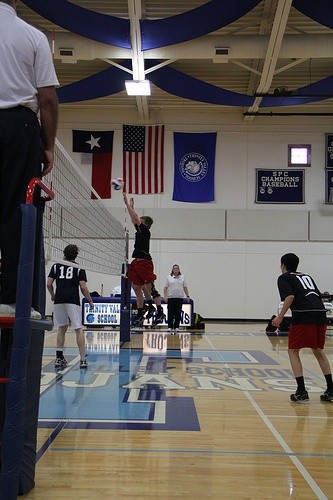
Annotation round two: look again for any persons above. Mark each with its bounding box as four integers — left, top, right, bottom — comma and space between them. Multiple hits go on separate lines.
122, 194, 164, 325
268, 252, 332, 401
0, 0, 62, 319
164, 264, 190, 332
47, 244, 94, 367
146, 285, 164, 324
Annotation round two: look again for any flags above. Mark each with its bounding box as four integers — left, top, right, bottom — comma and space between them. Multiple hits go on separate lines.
171, 130, 217, 204
71, 128, 114, 200
121, 123, 165, 195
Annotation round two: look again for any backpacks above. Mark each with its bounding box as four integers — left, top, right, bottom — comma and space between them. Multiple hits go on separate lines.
186, 313, 205, 330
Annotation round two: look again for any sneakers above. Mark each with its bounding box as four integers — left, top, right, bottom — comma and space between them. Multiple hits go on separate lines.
320, 392, 333, 402
290, 392, 309, 402
167, 328, 171, 332
0, 300, 41, 320
54, 356, 67, 366
80, 359, 88, 368
174, 328, 179, 332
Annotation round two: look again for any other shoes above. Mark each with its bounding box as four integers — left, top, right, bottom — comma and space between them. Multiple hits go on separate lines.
154, 312, 166, 325
146, 306, 156, 318
136, 305, 147, 316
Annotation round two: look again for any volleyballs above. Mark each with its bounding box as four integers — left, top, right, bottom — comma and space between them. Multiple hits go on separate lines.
112, 178, 124, 190
